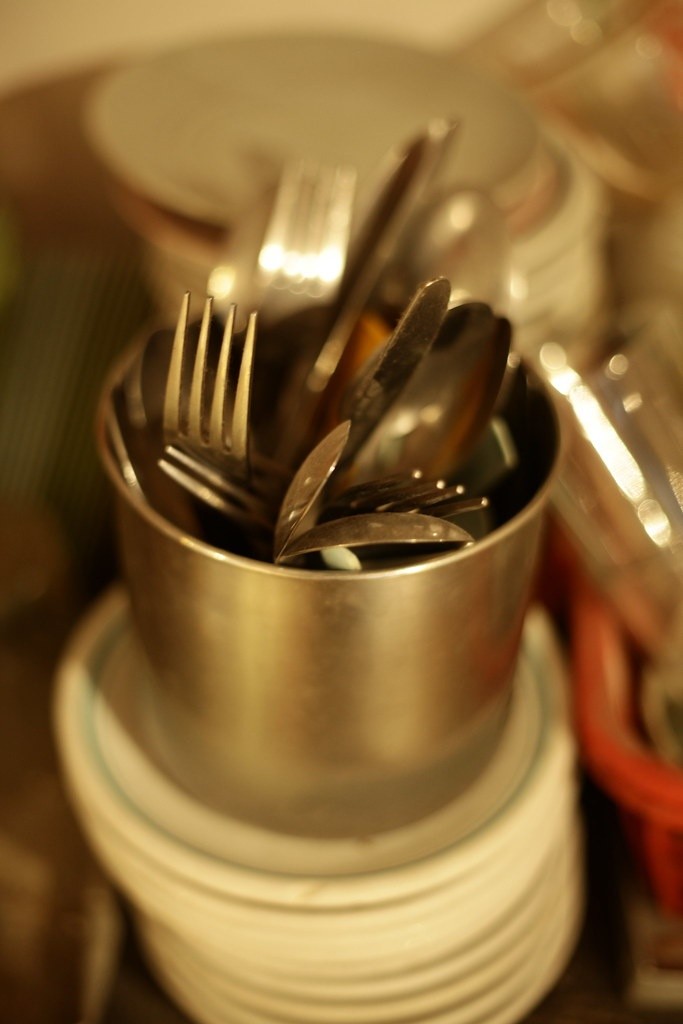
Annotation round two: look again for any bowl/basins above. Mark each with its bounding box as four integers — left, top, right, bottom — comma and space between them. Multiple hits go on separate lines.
570, 538, 680, 908
102, 157, 618, 390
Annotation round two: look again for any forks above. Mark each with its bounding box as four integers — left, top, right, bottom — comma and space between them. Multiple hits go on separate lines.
318, 467, 489, 515
247, 162, 355, 377
161, 293, 279, 542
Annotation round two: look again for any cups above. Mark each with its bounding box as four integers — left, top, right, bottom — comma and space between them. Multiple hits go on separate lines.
95, 290, 564, 836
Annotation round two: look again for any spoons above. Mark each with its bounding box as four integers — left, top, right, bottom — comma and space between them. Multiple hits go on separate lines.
339, 301, 518, 501
275, 420, 475, 569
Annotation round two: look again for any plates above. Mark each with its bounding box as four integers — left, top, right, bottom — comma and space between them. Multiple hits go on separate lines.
52, 579, 586, 1024
